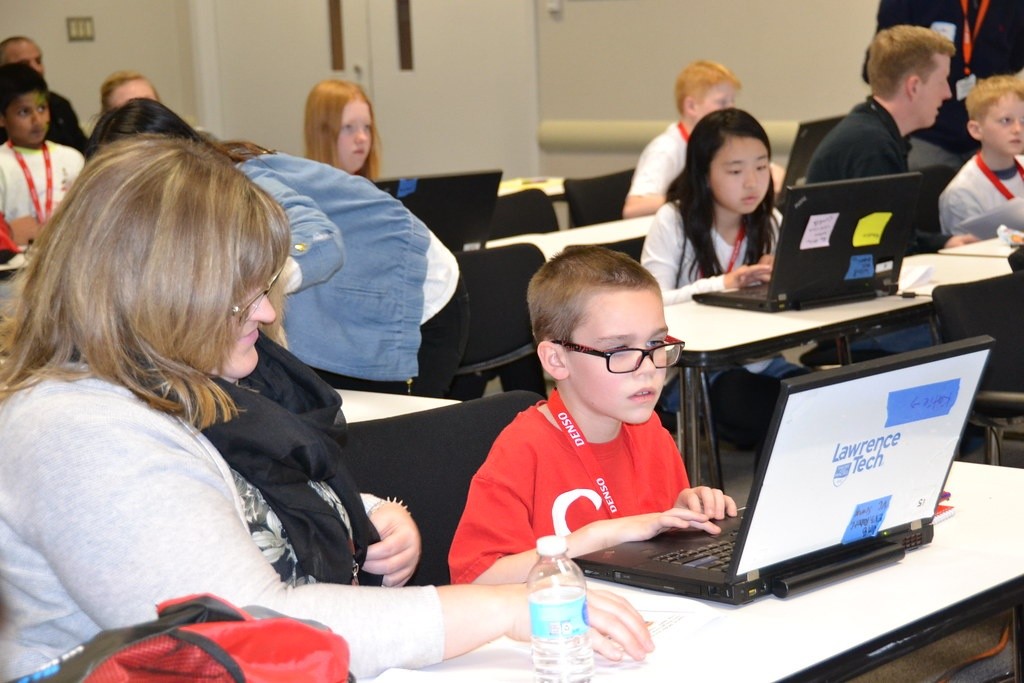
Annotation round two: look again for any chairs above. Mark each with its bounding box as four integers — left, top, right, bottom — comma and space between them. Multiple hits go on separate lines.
926, 276, 1024, 468
495, 189, 559, 238
561, 236, 646, 268
564, 167, 635, 226
449, 244, 548, 398
346, 392, 544, 587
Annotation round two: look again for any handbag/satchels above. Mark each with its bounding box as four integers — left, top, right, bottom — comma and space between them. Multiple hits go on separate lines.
8, 594, 358, 682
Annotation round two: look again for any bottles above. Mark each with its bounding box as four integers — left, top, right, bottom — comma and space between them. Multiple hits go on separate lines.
527, 535, 594, 683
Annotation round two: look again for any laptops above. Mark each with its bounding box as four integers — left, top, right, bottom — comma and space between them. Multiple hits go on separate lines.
571, 333, 996, 605
372, 171, 504, 254
691, 171, 924, 314
770, 115, 847, 212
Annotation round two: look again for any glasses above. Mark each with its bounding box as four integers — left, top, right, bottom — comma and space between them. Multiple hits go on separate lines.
226, 264, 284, 325
549, 335, 686, 375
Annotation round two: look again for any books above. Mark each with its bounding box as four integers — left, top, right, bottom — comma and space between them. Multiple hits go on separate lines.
930, 491, 956, 525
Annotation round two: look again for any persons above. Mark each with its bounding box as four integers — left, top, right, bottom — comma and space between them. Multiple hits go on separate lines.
367, 496, 411, 519
623, 60, 808, 379
304, 80, 381, 180
84, 98, 470, 398
0, 133, 653, 683
0, 35, 160, 245
806, 25, 956, 362
448, 245, 738, 584
862, 0, 1024, 190
939, 76, 1024, 241
641, 108, 793, 433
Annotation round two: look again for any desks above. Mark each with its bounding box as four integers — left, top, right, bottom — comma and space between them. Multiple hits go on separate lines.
331, 387, 461, 425
939, 236, 1023, 256
470, 213, 655, 258
893, 255, 1014, 296
661, 270, 944, 504
498, 176, 567, 199
379, 462, 1024, 683
